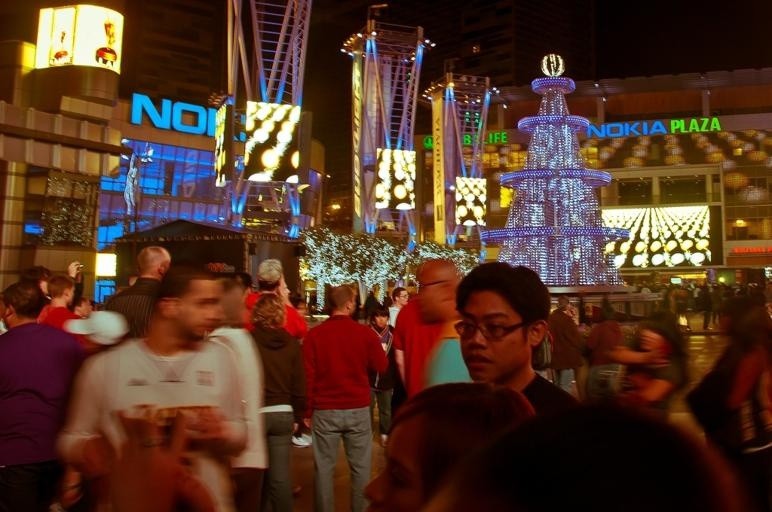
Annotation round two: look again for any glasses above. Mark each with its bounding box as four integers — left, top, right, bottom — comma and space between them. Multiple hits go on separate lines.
416, 280, 446, 288
453, 322, 527, 340
399, 294, 410, 298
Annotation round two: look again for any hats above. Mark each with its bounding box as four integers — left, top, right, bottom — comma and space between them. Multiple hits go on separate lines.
257, 258, 282, 283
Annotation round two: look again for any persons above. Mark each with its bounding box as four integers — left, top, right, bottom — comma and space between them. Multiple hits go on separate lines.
426, 407, 728, 511
623, 309, 690, 410
75, 299, 96, 319
584, 320, 680, 430
641, 273, 772, 328
243, 258, 308, 497
300, 284, 389, 512
387, 287, 409, 330
547, 296, 586, 399
454, 261, 582, 416
361, 383, 534, 511
21, 261, 85, 312
209, 288, 268, 512
37, 277, 90, 357
0, 283, 83, 512
249, 291, 307, 512
57, 269, 249, 511
370, 304, 393, 449
102, 246, 174, 347
683, 296, 772, 512
393, 258, 474, 398
282, 281, 290, 300
